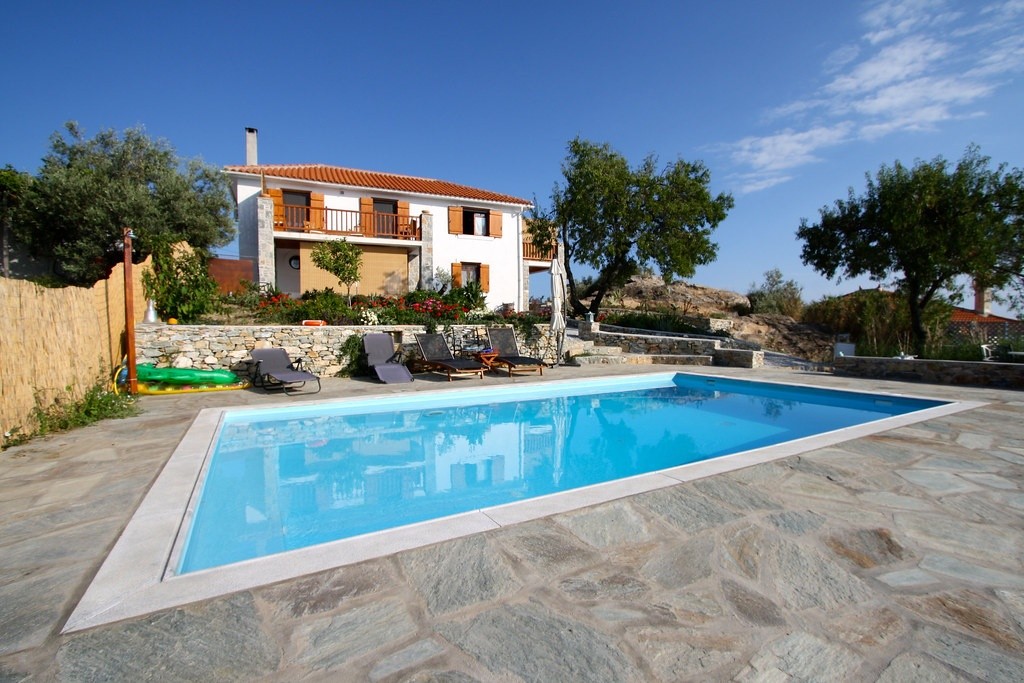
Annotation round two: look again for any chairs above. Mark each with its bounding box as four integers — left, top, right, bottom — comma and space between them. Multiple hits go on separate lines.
363, 331, 414, 383
249, 348, 322, 398
485, 327, 548, 377
413, 332, 490, 382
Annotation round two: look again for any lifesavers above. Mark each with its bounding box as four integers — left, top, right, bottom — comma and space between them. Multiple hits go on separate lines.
302, 320, 327, 326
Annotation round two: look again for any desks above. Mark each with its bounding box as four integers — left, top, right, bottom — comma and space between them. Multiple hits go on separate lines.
474, 353, 499, 376
